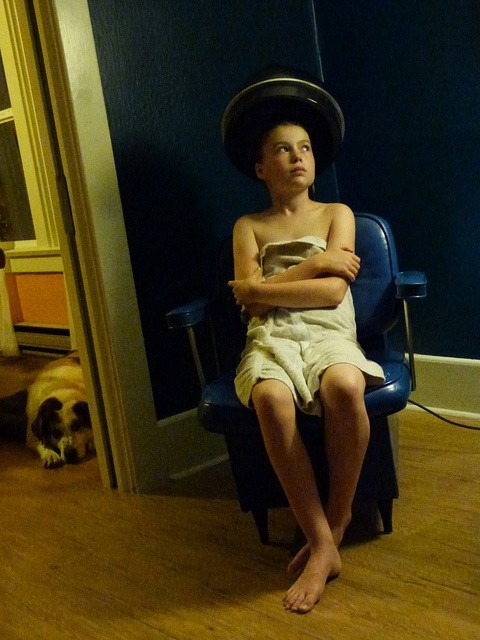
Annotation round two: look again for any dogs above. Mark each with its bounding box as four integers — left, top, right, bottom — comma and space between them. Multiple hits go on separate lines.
26, 356, 96, 469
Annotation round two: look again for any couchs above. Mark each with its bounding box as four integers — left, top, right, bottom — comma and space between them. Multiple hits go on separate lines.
171, 210, 430, 547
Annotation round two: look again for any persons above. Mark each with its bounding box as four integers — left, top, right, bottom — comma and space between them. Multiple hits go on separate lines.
228, 118, 385, 615
